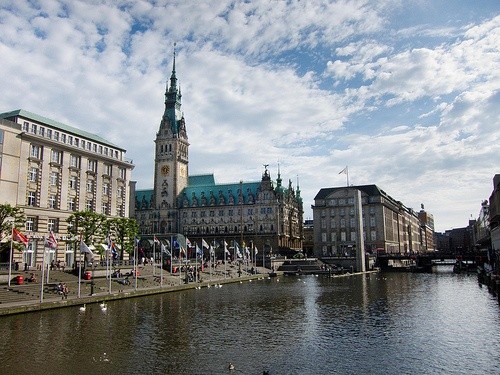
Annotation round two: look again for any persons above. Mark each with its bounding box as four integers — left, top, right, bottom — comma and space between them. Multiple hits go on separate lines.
51, 260, 60, 271
116, 269, 130, 285
173, 266, 196, 282
57, 283, 68, 299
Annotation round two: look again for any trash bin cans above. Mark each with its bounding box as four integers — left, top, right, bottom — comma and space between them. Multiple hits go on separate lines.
85, 272, 91, 279
17, 275, 24, 285
172, 267, 176, 273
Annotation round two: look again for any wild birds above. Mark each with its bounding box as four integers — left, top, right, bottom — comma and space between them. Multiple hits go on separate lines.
79, 303, 87, 312
194, 283, 223, 289
99, 301, 108, 312
239, 276, 306, 284
229, 362, 234, 371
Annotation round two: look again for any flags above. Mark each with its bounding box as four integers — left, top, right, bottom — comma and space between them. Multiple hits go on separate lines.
163, 245, 170, 255
173, 239, 179, 247
138, 243, 145, 257
202, 239, 209, 249
48, 231, 58, 248
187, 238, 192, 247
13, 227, 27, 249
112, 243, 118, 259
154, 236, 160, 245
81, 241, 93, 255
181, 247, 186, 256
196, 245, 202, 254
225, 241, 258, 258
